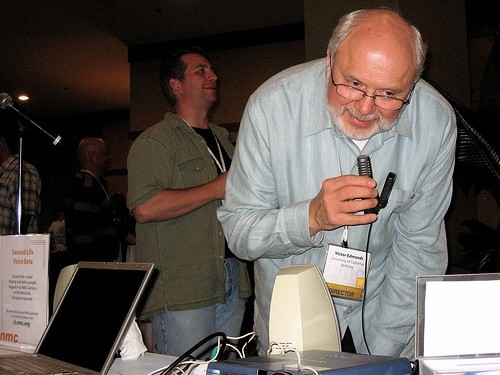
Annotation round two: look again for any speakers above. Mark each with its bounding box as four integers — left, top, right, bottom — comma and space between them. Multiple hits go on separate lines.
268, 265, 341, 353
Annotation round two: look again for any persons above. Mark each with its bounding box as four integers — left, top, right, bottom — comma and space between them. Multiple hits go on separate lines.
0, 125, 137, 265
217, 8, 457, 361
124, 47, 251, 360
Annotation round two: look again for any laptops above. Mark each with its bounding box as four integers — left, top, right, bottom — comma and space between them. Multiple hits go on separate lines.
0, 262, 155, 375
414, 272, 500, 375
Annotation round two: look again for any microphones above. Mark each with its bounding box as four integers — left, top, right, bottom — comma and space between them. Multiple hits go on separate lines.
0, 92, 63, 145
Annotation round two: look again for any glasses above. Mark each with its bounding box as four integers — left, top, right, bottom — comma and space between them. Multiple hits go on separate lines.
329, 50, 415, 110
